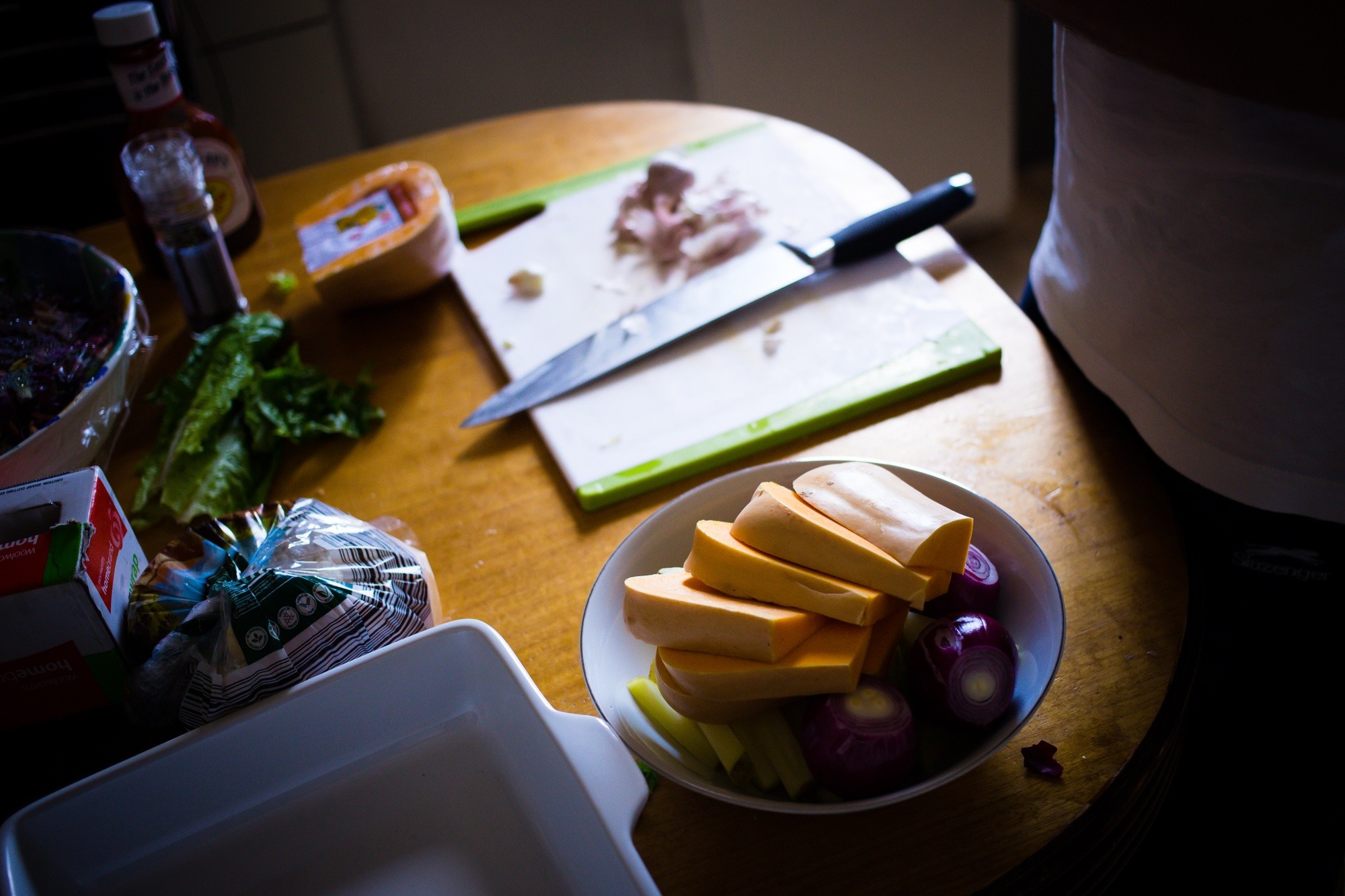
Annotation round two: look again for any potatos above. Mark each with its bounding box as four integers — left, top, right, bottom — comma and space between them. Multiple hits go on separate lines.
622, 462, 975, 727
292, 159, 460, 320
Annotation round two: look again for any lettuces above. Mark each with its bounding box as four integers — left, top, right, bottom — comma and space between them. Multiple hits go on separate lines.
133, 308, 389, 540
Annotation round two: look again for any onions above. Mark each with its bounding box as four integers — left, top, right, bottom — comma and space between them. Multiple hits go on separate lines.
798, 541, 1021, 788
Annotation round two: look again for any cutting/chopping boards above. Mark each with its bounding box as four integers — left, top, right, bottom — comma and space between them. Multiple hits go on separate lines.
444, 124, 999, 510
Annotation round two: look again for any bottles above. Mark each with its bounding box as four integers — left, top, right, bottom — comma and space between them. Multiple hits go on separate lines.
94, 2, 262, 276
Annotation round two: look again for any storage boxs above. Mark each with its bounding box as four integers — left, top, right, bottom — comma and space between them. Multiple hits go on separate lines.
0, 467, 151, 822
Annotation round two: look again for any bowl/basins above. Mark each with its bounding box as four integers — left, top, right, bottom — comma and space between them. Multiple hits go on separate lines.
1, 225, 137, 489
580, 455, 1066, 823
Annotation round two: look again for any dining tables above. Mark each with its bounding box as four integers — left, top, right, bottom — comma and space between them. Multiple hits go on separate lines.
67, 98, 1186, 896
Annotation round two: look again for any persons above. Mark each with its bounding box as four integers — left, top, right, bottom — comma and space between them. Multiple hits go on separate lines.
1010, 2, 1345, 896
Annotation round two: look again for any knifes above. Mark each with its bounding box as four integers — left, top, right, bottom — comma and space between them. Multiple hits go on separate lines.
463, 171, 977, 432
